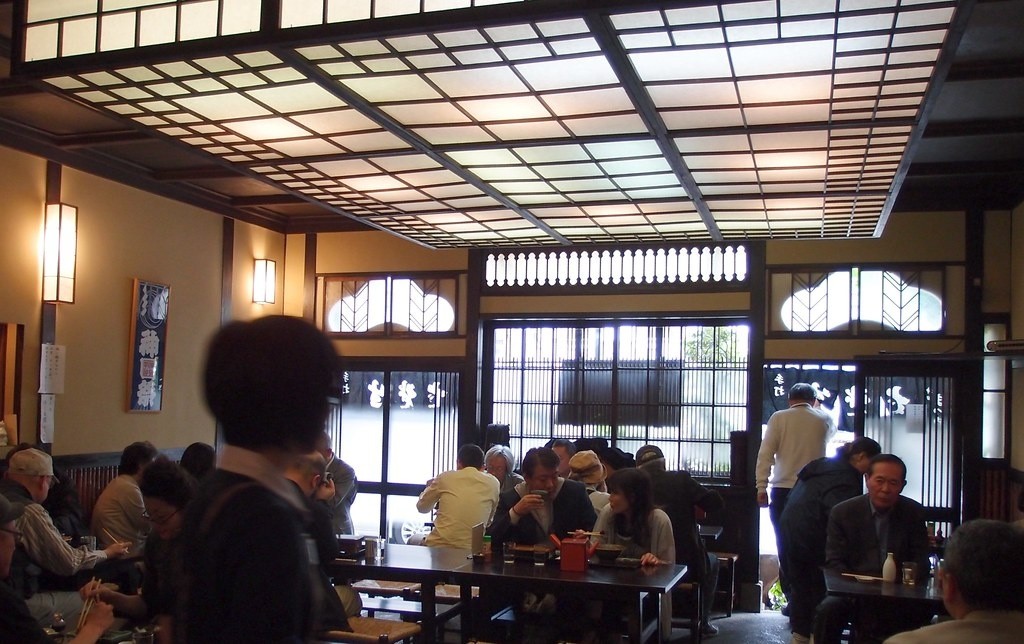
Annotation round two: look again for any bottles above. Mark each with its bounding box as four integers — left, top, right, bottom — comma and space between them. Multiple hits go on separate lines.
482, 536, 493, 561
52, 613, 66, 631
882, 553, 896, 582
365, 536, 377, 563
926, 522, 937, 543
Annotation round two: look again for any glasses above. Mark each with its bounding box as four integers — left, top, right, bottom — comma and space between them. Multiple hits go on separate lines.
323, 393, 339, 409
0, 526, 23, 542
139, 507, 180, 526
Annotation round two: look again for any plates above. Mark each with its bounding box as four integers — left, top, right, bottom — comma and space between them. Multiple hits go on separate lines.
41, 628, 58, 634
856, 576, 876, 582
67, 629, 131, 641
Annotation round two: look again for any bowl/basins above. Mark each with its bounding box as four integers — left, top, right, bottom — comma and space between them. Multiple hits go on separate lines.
595, 544, 625, 562
472, 554, 484, 563
531, 489, 548, 502
514, 546, 551, 556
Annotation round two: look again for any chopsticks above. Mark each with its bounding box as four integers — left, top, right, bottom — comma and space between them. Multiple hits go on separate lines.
843, 572, 888, 579
77, 576, 101, 629
101, 527, 130, 554
568, 531, 604, 535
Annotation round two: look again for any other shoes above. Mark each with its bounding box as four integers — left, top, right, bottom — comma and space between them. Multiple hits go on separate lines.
700, 620, 718, 637
792, 632, 809, 644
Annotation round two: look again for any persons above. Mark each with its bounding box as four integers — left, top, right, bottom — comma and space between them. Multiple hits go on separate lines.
756, 383, 837, 616
415, 439, 719, 643
1, 431, 358, 644
814, 454, 925, 644
882, 519, 1024, 644
163, 312, 346, 644
780, 437, 881, 644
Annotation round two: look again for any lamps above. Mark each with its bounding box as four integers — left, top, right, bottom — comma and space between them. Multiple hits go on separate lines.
42, 202, 78, 304
252, 259, 275, 305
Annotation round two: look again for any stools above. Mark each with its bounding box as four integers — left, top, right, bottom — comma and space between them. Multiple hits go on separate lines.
708, 551, 740, 618
320, 579, 479, 644
673, 583, 702, 644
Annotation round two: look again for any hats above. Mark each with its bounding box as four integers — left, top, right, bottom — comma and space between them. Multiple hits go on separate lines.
566, 449, 607, 484
0, 494, 25, 525
9, 449, 61, 484
788, 382, 815, 401
635, 446, 665, 470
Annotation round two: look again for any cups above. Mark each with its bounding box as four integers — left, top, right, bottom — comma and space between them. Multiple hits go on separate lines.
902, 562, 916, 585
81, 536, 96, 550
503, 542, 514, 563
534, 544, 547, 565
133, 632, 154, 644
373, 539, 385, 560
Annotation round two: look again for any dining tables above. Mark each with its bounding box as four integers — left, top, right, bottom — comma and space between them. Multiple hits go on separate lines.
333, 544, 688, 644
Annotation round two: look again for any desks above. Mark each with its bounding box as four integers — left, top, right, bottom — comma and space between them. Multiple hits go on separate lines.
821, 567, 950, 644
691, 526, 723, 644
